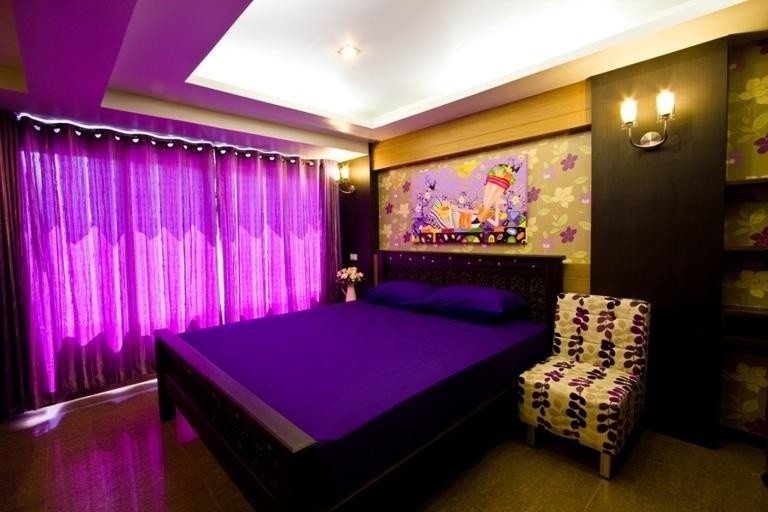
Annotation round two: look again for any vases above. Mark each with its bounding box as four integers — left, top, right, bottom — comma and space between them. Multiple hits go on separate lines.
340, 281, 357, 302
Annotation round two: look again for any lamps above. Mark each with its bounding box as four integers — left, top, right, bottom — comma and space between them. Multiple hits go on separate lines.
618, 91, 676, 149
330, 165, 354, 195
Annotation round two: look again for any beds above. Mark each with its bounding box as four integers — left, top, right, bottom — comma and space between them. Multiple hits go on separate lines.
152, 248, 566, 512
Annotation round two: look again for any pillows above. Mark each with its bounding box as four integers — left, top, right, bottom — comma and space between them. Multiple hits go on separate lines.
425, 285, 523, 322
365, 278, 443, 310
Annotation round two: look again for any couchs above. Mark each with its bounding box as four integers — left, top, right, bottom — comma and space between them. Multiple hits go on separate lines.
519, 292, 651, 481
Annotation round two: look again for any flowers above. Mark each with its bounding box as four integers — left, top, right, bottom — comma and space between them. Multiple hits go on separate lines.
332, 267, 364, 284
